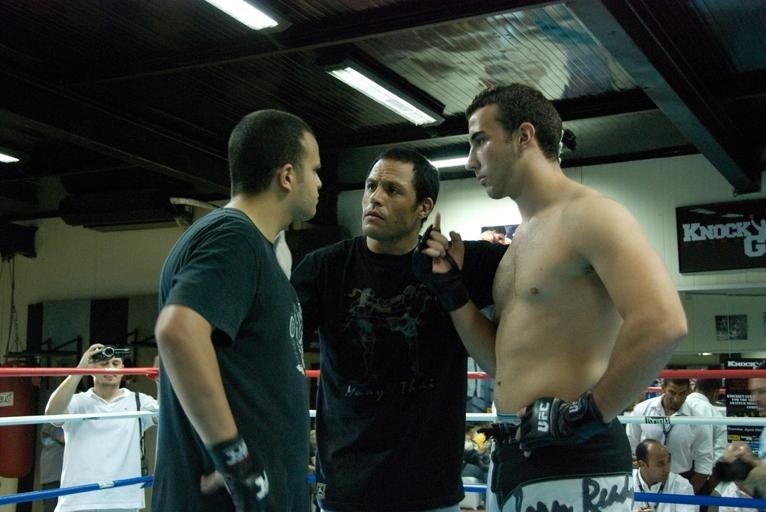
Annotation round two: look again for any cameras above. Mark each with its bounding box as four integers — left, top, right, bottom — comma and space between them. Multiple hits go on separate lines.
93, 346, 131, 361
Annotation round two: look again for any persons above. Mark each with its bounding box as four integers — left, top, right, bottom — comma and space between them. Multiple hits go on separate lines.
710, 440, 766, 499
289, 144, 517, 511
461, 418, 497, 482
303, 419, 325, 511
746, 361, 766, 460
631, 435, 702, 512
678, 361, 727, 511
409, 82, 692, 511
621, 365, 715, 496
43, 341, 162, 512
481, 225, 510, 246
152, 107, 324, 512
39, 422, 64, 511
